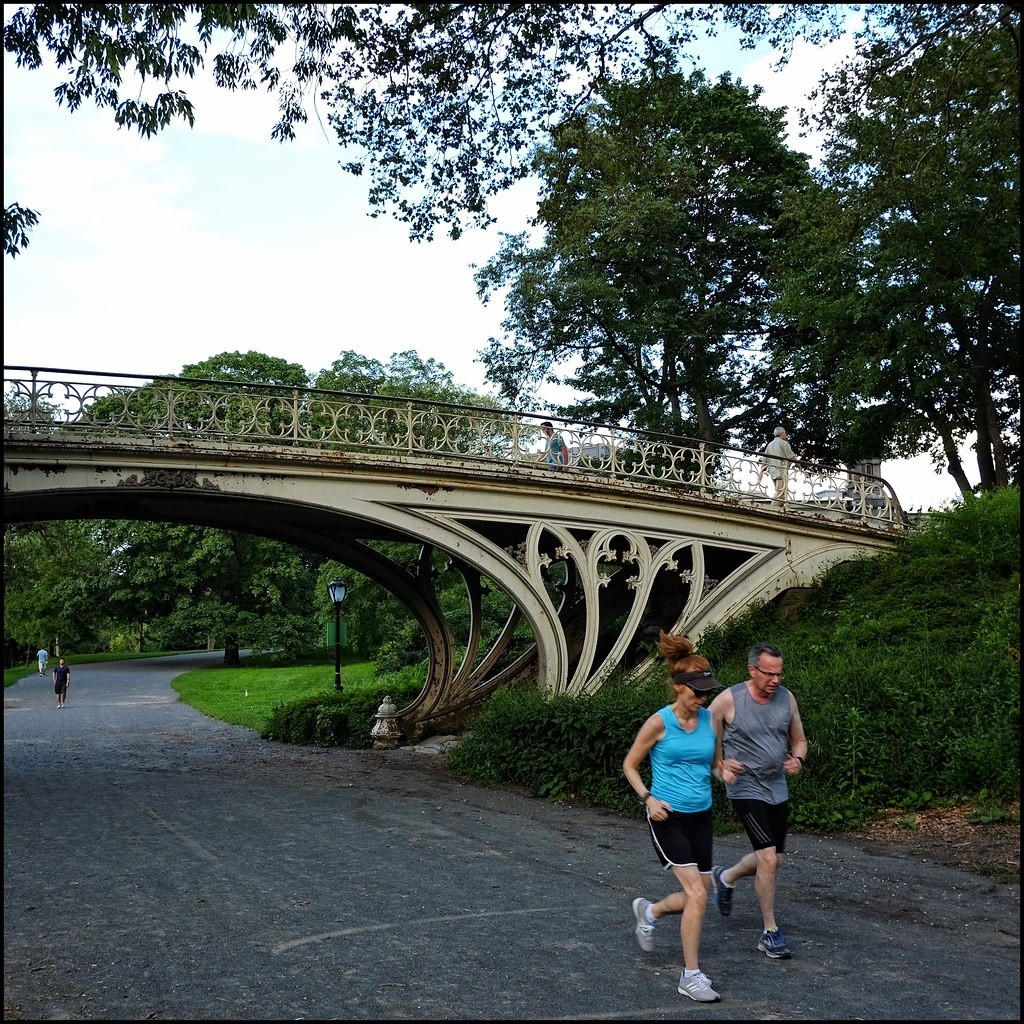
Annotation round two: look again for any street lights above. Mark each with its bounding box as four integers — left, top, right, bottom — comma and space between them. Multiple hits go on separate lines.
327, 576, 348, 691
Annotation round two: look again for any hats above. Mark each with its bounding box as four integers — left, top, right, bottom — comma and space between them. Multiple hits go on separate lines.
673, 669, 726, 691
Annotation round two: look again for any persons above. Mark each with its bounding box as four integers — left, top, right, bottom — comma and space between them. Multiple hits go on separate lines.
622, 629, 737, 1003
707, 643, 808, 959
536, 421, 569, 473
53, 658, 70, 709
36, 646, 49, 677
758, 426, 802, 508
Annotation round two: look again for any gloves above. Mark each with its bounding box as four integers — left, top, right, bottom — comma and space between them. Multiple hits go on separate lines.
46, 661, 49, 664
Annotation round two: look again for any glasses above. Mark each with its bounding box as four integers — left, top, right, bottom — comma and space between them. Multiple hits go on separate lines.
683, 683, 714, 697
752, 666, 787, 679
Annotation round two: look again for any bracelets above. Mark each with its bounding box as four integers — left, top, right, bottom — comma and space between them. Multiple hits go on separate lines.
641, 792, 652, 803
795, 757, 806, 767
67, 681, 69, 683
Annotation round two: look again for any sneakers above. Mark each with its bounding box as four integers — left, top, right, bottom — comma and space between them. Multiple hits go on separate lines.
677, 971, 721, 1002
710, 865, 735, 917
632, 897, 657, 952
757, 930, 792, 960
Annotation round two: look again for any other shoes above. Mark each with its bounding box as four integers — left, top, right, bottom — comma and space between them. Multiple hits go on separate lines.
57, 705, 65, 709
39, 674, 44, 677
